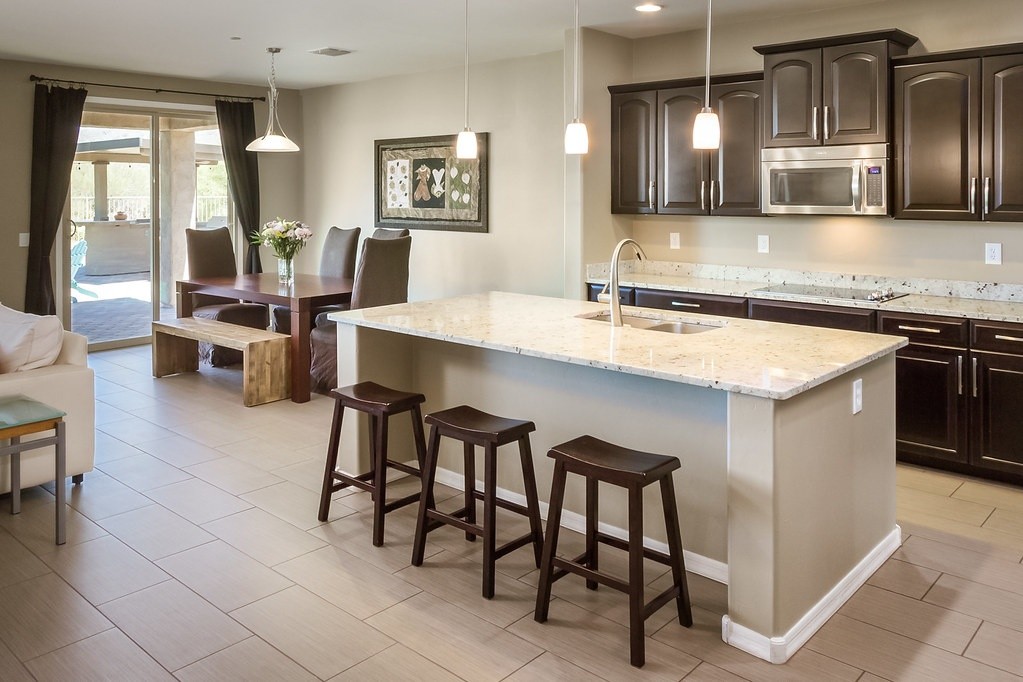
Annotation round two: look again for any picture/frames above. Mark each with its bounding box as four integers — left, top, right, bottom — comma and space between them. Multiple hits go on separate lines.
371, 131, 491, 233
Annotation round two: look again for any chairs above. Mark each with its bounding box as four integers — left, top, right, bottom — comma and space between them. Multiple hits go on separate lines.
310, 235, 413, 393
71, 239, 99, 303
272, 227, 360, 334
184, 225, 267, 368
272, 227, 411, 335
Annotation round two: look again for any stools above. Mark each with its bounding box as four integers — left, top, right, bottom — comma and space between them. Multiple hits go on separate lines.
402, 402, 544, 600
312, 378, 426, 545
533, 433, 693, 668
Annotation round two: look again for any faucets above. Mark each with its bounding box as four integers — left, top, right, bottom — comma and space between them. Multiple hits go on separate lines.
610, 238, 647, 327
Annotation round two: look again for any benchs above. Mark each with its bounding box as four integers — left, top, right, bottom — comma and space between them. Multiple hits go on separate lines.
152, 319, 291, 407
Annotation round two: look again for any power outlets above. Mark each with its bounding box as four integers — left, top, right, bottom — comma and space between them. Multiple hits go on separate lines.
669, 232, 682, 251
985, 243, 1003, 264
851, 379, 867, 415
757, 234, 770, 253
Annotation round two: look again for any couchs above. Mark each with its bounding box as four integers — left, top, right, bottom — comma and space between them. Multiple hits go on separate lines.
0, 299, 98, 498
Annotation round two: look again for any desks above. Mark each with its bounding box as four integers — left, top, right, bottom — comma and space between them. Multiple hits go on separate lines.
170, 267, 354, 404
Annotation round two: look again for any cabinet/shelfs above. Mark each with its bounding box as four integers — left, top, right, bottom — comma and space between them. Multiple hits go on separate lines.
588, 284, 1022, 483
753, 30, 920, 145
606, 86, 658, 214
886, 44, 1023, 223
655, 72, 764, 218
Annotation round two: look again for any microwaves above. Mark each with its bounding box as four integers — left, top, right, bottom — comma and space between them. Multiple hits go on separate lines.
759, 143, 894, 217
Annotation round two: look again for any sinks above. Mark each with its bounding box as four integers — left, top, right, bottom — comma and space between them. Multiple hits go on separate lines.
585, 313, 661, 330
644, 322, 723, 334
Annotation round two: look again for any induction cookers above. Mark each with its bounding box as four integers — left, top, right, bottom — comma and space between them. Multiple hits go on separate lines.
750, 283, 908, 310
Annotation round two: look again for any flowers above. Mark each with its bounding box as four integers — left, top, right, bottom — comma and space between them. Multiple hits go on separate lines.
253, 212, 311, 253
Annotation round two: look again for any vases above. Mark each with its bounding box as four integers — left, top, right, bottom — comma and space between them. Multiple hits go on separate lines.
279, 251, 295, 279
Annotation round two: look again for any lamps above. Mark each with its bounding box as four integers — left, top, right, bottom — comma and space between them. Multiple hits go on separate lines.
456, 1, 477, 161
693, 1, 720, 149
560, 0, 591, 155
246, 48, 299, 153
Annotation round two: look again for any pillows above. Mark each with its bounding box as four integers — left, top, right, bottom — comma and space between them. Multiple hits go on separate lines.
0, 303, 66, 372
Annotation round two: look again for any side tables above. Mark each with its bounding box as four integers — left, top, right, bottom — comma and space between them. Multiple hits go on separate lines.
0, 397, 70, 555
